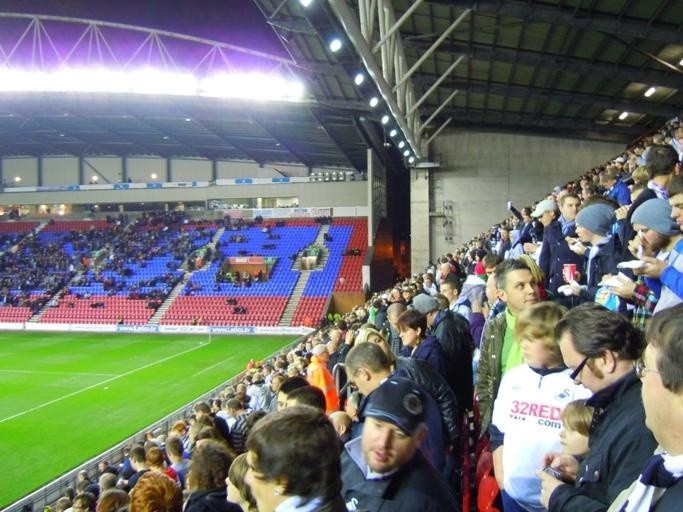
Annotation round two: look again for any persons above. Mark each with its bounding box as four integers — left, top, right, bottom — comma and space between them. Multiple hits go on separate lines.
1, 117, 682, 512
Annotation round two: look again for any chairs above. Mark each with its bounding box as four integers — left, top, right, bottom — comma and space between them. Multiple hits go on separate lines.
462, 391, 501, 512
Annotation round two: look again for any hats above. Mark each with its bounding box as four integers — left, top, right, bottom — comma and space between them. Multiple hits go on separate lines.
410, 293, 441, 316
529, 199, 558, 220
360, 375, 429, 439
630, 198, 680, 238
574, 203, 616, 239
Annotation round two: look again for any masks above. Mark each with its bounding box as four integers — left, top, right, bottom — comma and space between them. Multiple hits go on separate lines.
527, 365, 568, 379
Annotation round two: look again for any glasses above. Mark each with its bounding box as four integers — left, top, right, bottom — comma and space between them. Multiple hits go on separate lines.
634, 355, 673, 383
570, 356, 589, 383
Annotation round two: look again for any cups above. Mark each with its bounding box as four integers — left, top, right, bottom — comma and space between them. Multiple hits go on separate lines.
563, 263, 576, 284
